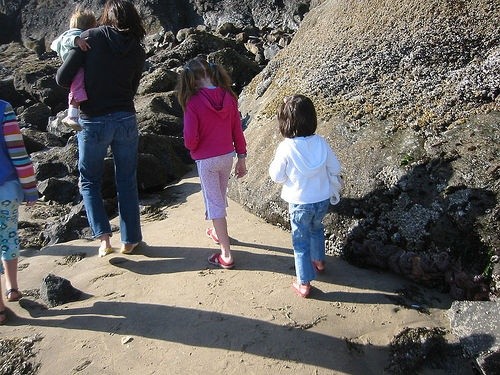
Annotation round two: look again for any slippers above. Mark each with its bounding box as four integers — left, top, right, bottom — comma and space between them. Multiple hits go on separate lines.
208, 254, 235, 268
206, 228, 220, 243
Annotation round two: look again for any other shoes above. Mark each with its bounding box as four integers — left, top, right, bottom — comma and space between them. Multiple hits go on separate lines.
120, 242, 138, 253
293, 280, 310, 295
312, 259, 325, 271
98, 247, 112, 255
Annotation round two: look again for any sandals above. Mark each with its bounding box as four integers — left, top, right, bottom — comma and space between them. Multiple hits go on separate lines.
7, 288, 22, 301
0, 308, 7, 324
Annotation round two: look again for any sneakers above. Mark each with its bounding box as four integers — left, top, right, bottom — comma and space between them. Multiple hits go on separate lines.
61, 117, 82, 131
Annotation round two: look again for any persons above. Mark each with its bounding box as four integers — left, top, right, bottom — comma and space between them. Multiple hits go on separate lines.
56, 10, 97, 132
0, 98, 38, 326
178, 57, 248, 269
55, 0, 147, 257
269, 94, 342, 298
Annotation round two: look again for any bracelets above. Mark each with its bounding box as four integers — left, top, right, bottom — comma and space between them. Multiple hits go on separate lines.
237, 153, 247, 159
